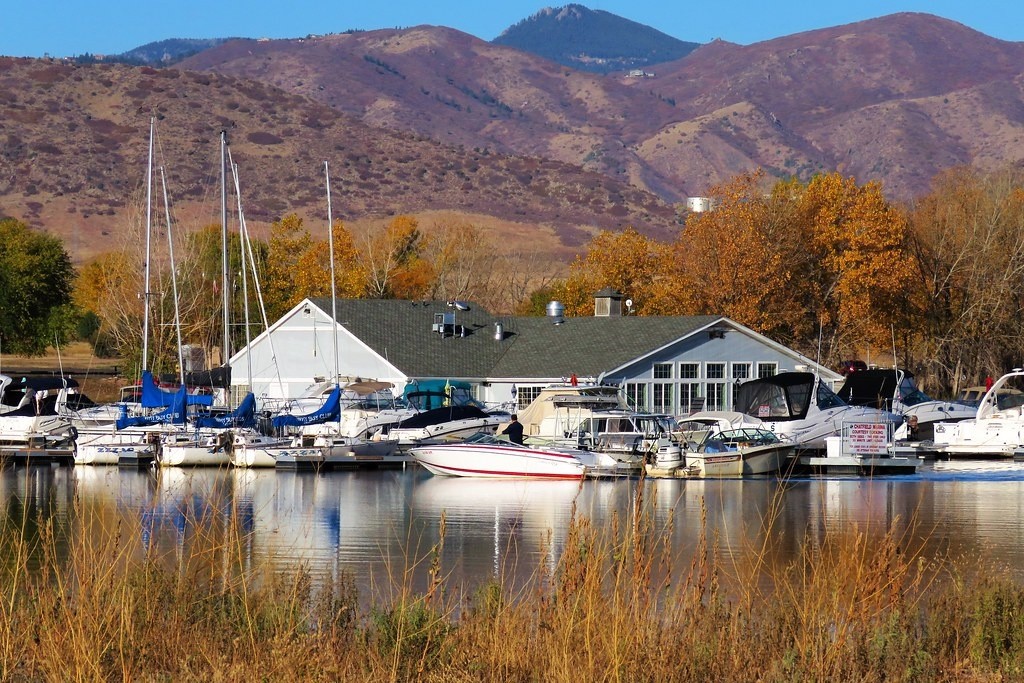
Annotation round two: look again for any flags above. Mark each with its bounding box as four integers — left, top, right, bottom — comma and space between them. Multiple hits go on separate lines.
893, 421, 908, 440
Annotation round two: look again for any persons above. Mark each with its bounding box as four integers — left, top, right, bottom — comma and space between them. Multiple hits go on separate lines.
984, 375, 993, 393
570, 372, 578, 387
511, 383, 517, 397
496, 414, 526, 446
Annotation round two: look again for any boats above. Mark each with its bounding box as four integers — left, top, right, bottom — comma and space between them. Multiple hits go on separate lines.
1, 115, 1024, 483
